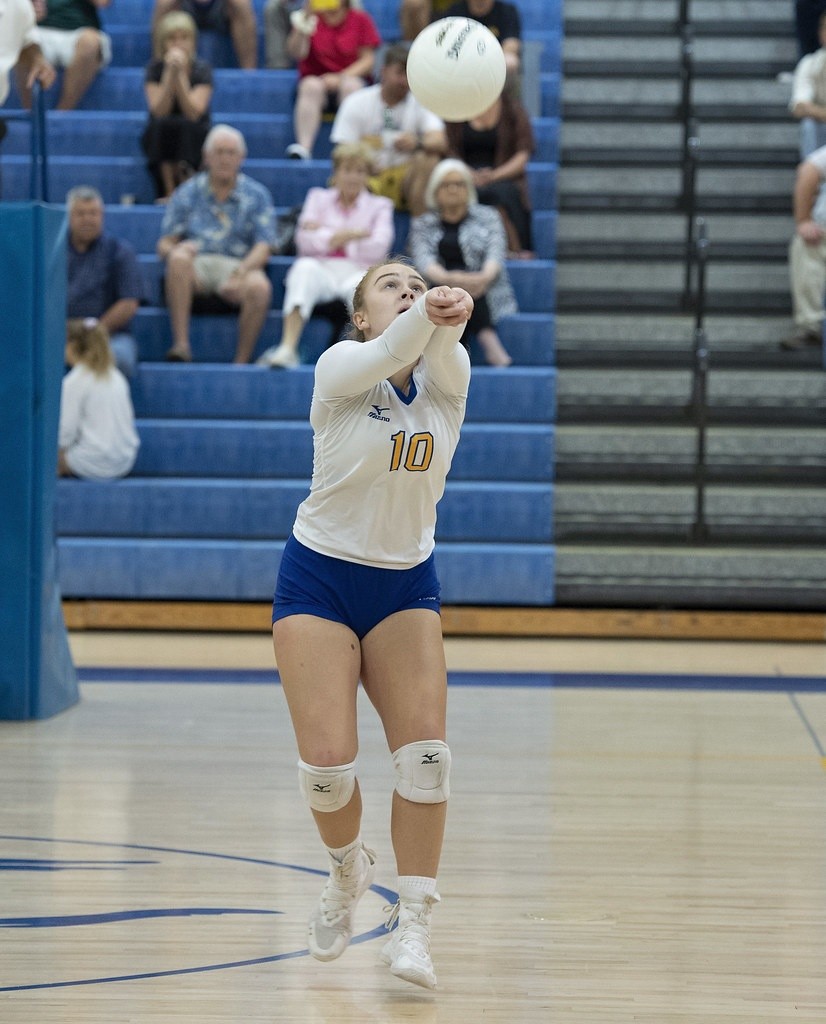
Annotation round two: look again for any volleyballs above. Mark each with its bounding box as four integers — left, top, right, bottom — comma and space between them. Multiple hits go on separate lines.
406, 16, 506, 122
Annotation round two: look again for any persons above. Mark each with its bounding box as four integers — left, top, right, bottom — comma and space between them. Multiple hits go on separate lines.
782, 0, 826, 350
0, 0, 114, 142
271, 259, 472, 991
57, 184, 149, 480
139, 0, 258, 205
155, 123, 275, 364
262, 0, 536, 369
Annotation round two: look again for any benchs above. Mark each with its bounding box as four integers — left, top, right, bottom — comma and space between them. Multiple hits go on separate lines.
0, 0, 562, 610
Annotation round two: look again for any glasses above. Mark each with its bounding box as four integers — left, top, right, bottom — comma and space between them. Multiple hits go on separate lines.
440, 181, 466, 188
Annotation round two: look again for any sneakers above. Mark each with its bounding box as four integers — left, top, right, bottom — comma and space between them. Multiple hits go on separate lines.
380, 887, 441, 991
307, 841, 378, 963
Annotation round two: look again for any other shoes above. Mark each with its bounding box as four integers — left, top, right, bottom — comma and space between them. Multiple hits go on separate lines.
284, 144, 309, 161
165, 352, 192, 363
270, 347, 300, 370
780, 330, 824, 351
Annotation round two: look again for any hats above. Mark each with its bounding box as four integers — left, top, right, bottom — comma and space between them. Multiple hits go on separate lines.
310, 0, 340, 11
